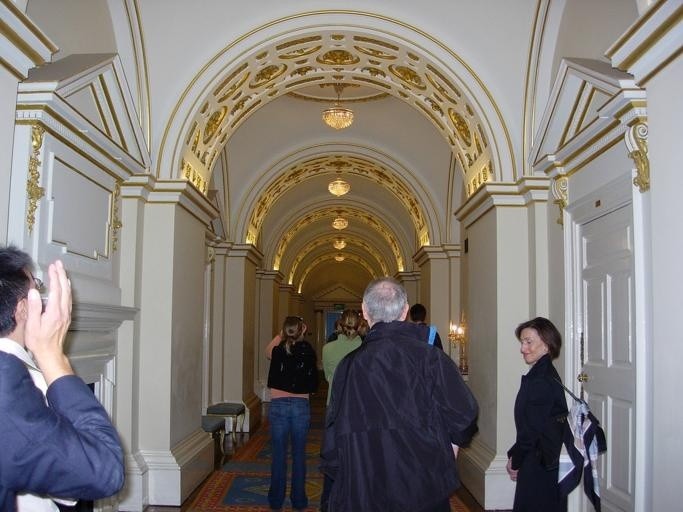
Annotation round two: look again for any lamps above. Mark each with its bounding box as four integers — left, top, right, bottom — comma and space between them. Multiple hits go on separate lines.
322, 85, 356, 133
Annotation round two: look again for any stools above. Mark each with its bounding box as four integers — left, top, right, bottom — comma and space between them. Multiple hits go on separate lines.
203, 400, 246, 456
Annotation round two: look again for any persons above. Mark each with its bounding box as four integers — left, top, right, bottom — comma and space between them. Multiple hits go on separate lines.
410, 304, 443, 351
322, 309, 363, 406
327, 319, 342, 343
358, 318, 369, 342
0, 241, 125, 512
506, 316, 569, 512
319, 276, 479, 511
265, 316, 318, 510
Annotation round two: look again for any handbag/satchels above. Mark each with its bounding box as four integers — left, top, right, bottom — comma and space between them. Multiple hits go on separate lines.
538, 404, 607, 472
